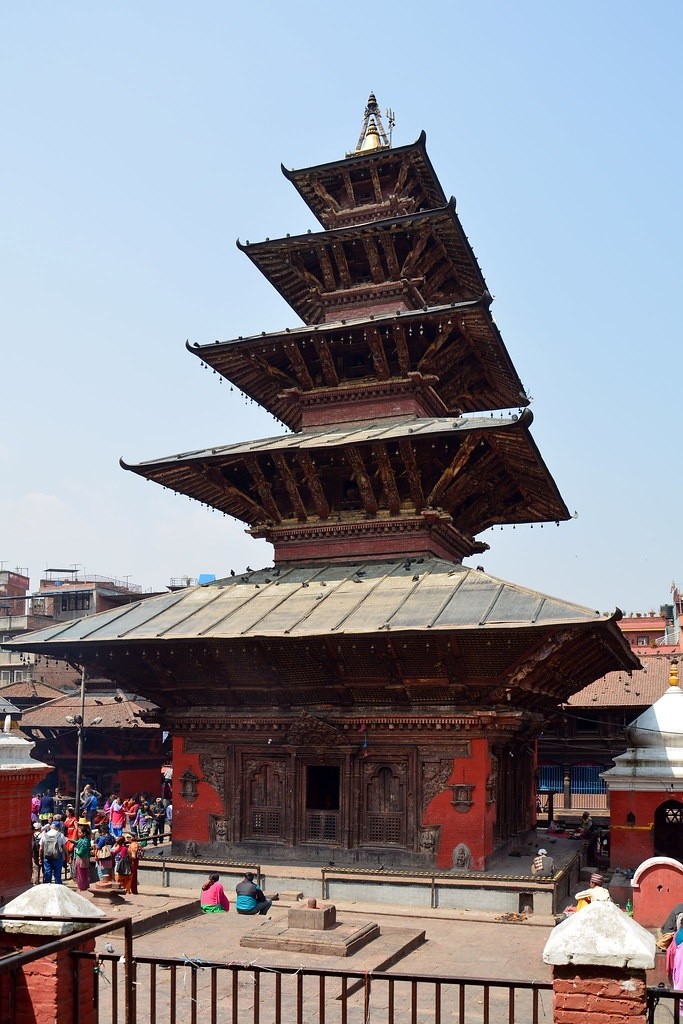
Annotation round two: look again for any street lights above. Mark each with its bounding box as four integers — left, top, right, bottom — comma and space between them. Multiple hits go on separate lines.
65, 715, 104, 880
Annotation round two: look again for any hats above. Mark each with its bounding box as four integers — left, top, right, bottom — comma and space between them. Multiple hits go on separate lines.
591, 874, 605, 885
584, 811, 590, 816
75, 818, 91, 825
52, 821, 61, 829
538, 849, 547, 856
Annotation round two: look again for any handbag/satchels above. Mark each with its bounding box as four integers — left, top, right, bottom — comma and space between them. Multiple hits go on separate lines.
137, 845, 144, 859
97, 844, 111, 860
116, 859, 131, 874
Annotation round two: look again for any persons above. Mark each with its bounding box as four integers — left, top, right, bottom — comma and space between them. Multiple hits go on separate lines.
200, 874, 229, 913
531, 849, 557, 877
31, 784, 172, 894
236, 872, 272, 915
575, 874, 611, 911
656, 902, 683, 1017
457, 848, 465, 867
580, 812, 594, 836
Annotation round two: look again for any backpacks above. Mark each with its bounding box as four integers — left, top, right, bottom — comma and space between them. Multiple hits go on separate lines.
43, 832, 61, 859
33, 832, 41, 858
